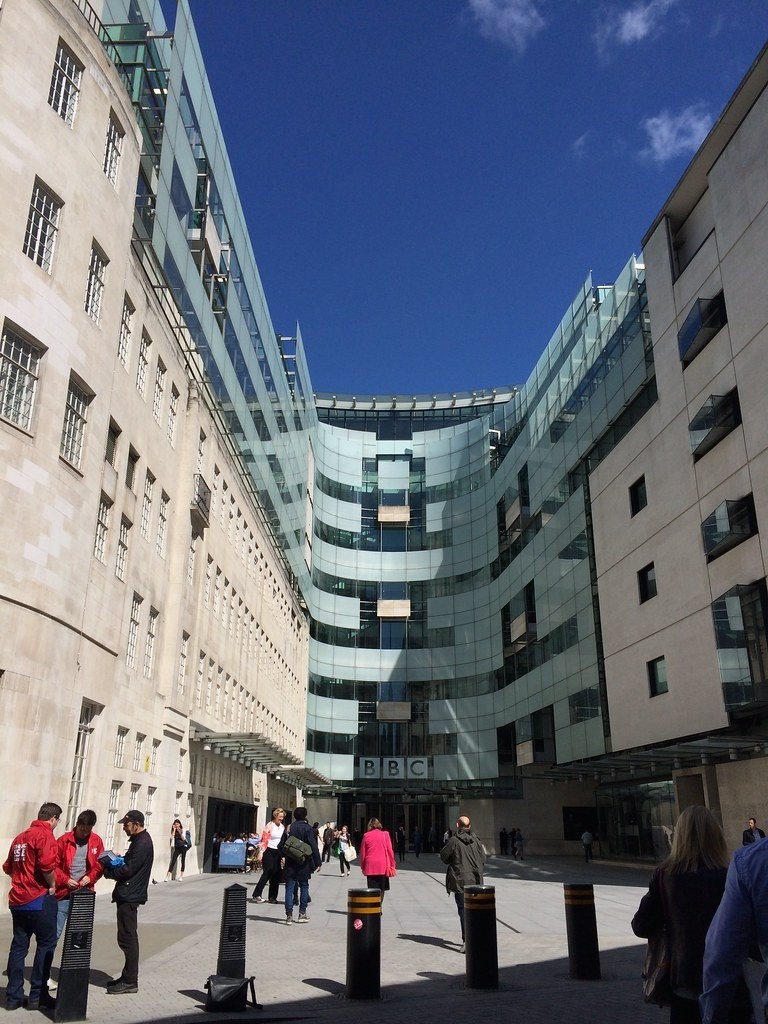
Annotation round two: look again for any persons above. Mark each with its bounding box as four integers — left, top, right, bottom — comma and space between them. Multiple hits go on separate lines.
443, 827, 452, 844
321, 822, 338, 863
164, 819, 188, 882
312, 822, 324, 847
103, 810, 155, 992
3, 802, 63, 1011
509, 828, 524, 860
441, 818, 487, 953
698, 838, 768, 1024
631, 805, 728, 1024
276, 807, 321, 925
252, 808, 286, 904
360, 817, 397, 916
48, 810, 103, 991
213, 830, 259, 874
742, 817, 766, 846
581, 829, 593, 861
428, 827, 438, 853
395, 823, 405, 862
500, 828, 508, 855
335, 825, 352, 877
411, 825, 423, 858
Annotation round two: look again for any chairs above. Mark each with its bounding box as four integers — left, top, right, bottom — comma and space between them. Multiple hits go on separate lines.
246, 844, 262, 873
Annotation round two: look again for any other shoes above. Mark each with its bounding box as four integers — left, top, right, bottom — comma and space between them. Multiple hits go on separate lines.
340, 873, 344, 877
178, 878, 182, 881
287, 916, 292, 925
347, 869, 350, 875
164, 877, 169, 881
307, 896, 311, 902
461, 943, 466, 953
299, 914, 310, 922
292, 899, 299, 905
47, 978, 59, 990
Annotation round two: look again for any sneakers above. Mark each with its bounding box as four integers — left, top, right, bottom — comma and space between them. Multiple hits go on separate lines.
252, 895, 265, 903
6, 995, 28, 1010
107, 977, 121, 986
268, 897, 278, 903
28, 994, 57, 1009
107, 980, 138, 993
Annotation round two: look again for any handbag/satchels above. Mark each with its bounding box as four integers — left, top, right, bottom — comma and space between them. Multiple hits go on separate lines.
642, 933, 688, 1004
177, 832, 191, 850
282, 836, 312, 865
343, 847, 357, 862
386, 866, 396, 877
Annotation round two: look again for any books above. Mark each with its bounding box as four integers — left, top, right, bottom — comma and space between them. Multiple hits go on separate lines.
97, 849, 126, 868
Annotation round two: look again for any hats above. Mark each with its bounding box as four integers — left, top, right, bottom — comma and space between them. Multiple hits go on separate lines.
118, 810, 144, 823
